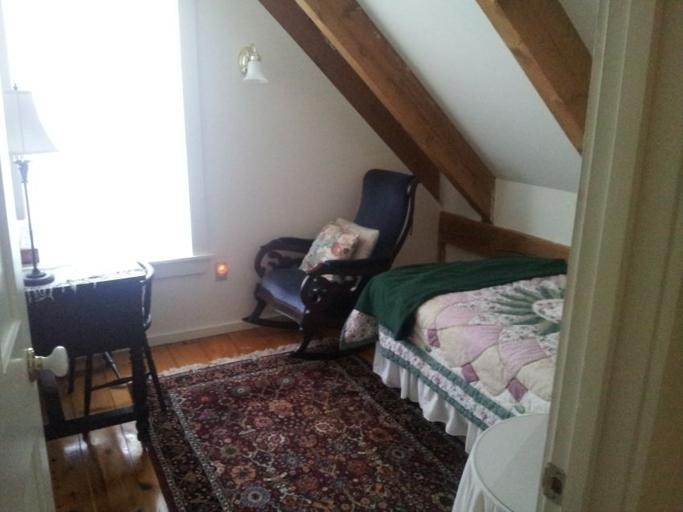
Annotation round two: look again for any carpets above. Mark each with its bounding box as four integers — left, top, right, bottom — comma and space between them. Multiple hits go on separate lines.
126, 336, 470, 512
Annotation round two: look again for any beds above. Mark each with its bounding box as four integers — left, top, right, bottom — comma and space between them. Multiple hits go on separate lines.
372, 252, 571, 455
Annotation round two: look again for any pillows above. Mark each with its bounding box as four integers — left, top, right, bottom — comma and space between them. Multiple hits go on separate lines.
334, 217, 381, 283
298, 221, 360, 282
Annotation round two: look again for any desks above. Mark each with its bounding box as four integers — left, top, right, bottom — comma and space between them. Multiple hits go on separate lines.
21, 252, 152, 442
451, 414, 549, 512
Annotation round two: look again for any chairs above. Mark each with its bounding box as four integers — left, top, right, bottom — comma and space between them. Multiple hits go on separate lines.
242, 169, 422, 359
64, 259, 168, 440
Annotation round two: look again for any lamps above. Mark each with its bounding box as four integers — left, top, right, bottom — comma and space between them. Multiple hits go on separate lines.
237, 44, 269, 84
3, 84, 57, 286
